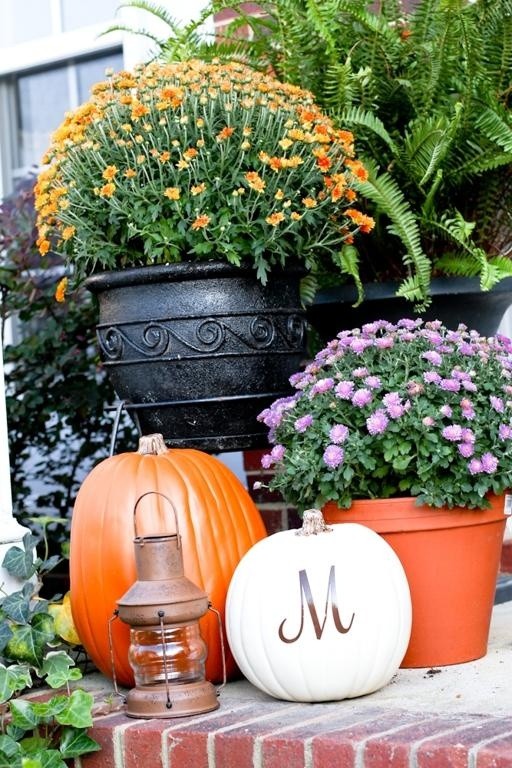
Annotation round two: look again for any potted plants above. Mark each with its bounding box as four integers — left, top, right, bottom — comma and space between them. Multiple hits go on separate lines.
98, 0, 512, 338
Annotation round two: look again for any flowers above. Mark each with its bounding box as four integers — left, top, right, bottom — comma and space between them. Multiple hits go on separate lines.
256, 319, 512, 512
34, 59, 377, 300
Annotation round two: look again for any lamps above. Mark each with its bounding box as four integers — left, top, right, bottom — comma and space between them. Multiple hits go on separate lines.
107, 491, 227, 719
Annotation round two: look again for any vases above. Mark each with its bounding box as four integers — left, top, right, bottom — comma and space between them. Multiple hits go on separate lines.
84, 261, 305, 452
319, 490, 512, 668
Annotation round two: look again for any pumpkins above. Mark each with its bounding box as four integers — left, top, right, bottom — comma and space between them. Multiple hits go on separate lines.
221, 511, 415, 704
65, 432, 271, 687
